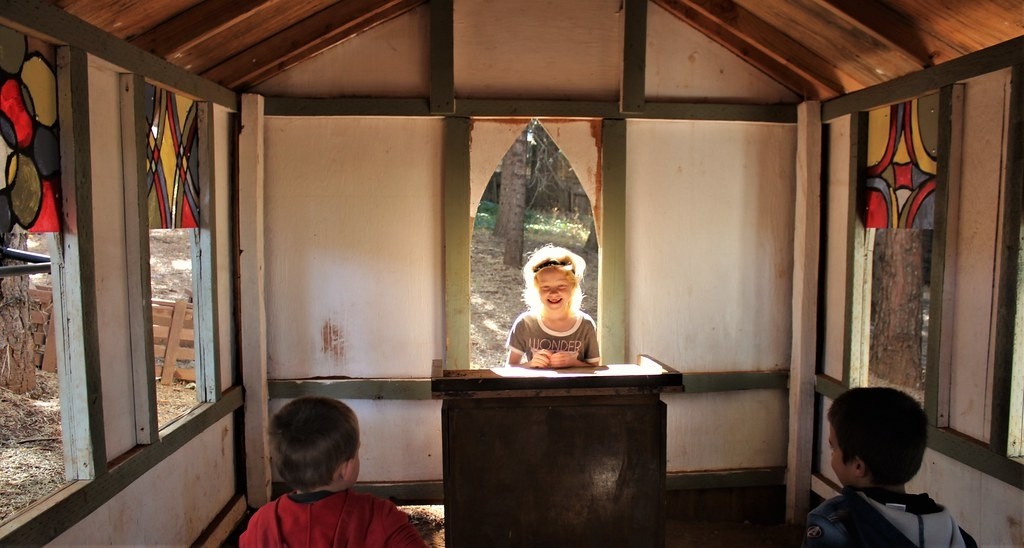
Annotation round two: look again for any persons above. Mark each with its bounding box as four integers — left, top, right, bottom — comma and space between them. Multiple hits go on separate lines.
238, 396, 428, 548
504, 248, 600, 369
805, 387, 979, 548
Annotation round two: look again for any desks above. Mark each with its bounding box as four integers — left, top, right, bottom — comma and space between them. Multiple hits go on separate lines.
431, 354, 684, 548
